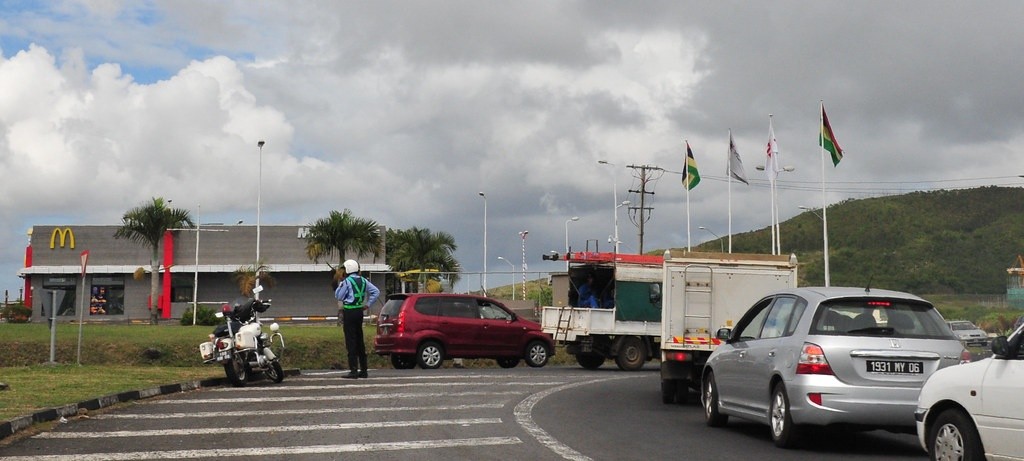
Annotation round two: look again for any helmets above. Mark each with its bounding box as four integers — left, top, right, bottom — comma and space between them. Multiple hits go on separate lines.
341, 259, 360, 274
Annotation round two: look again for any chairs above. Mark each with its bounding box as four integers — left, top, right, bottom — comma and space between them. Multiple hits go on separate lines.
824, 312, 916, 335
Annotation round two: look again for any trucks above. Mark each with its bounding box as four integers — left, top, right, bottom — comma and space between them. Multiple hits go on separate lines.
539, 250, 669, 373
659, 248, 799, 410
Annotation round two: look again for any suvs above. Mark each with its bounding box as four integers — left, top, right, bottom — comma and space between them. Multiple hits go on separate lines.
373, 291, 557, 371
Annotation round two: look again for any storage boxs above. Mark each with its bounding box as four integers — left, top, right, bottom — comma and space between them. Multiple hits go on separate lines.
200, 342, 214, 359
234, 332, 257, 348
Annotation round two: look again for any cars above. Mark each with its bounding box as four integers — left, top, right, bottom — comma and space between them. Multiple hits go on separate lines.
913, 322, 1024, 461
699, 285, 971, 448
946, 320, 992, 345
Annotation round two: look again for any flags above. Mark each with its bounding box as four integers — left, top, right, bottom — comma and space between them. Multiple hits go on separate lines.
681, 144, 700, 190
818, 104, 845, 167
763, 120, 779, 186
726, 134, 749, 185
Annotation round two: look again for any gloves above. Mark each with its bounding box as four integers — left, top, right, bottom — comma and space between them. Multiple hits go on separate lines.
363, 305, 368, 310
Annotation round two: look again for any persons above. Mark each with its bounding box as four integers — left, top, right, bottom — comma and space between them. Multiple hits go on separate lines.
334, 259, 380, 379
577, 274, 599, 308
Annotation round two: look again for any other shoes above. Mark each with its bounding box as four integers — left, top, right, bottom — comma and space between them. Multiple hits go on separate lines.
342, 371, 358, 379
358, 369, 367, 378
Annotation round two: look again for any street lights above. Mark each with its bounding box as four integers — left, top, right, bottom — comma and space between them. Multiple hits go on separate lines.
30, 286, 34, 310
564, 215, 580, 258
498, 256, 516, 301
598, 159, 630, 255
756, 164, 798, 258
478, 192, 488, 319
255, 140, 264, 323
798, 206, 835, 289
698, 226, 726, 257
518, 230, 531, 300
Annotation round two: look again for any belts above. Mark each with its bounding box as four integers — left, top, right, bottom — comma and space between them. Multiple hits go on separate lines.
344, 305, 363, 309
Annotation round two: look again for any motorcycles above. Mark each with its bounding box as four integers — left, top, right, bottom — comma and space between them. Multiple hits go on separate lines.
198, 297, 285, 387
